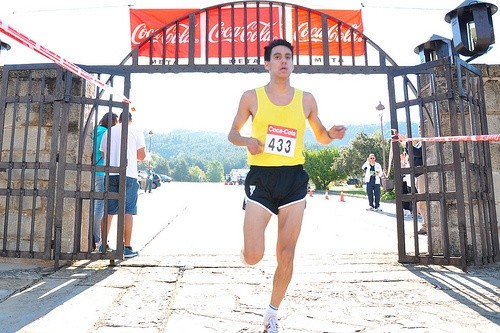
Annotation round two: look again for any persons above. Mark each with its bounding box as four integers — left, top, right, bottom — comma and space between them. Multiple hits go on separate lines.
400, 124, 427, 235
98, 110, 148, 258
144, 166, 155, 193
227, 174, 243, 185
227, 39, 349, 333
362, 154, 383, 212
92, 111, 118, 250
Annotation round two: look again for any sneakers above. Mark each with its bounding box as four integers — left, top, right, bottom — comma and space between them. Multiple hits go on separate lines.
264, 314, 279, 333
124, 247, 138, 258
99, 244, 112, 253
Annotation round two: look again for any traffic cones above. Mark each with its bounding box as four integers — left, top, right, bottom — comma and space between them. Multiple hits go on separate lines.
324, 189, 329, 199
339, 190, 346, 202
309, 191, 314, 197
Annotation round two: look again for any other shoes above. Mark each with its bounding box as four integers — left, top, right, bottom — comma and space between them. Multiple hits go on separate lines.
418, 215, 422, 219
375, 208, 382, 212
366, 207, 374, 211
417, 229, 427, 234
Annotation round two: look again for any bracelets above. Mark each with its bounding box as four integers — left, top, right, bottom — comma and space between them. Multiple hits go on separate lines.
327, 132, 335, 139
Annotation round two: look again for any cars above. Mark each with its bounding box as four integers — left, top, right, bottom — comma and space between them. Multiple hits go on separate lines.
138, 173, 173, 190
224, 168, 251, 185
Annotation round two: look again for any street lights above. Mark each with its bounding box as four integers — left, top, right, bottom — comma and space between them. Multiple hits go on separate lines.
148, 130, 154, 169
375, 100, 385, 174
